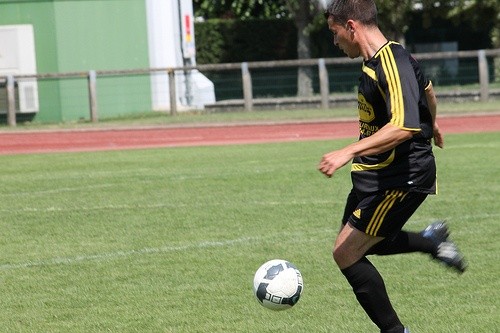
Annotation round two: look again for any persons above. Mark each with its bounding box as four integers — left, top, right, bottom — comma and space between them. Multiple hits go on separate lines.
317, 0, 468, 333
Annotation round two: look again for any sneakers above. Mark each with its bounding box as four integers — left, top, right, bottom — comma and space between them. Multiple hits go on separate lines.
421, 222, 466, 274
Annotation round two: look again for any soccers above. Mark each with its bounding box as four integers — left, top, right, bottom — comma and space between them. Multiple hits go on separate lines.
253, 259, 304, 311
